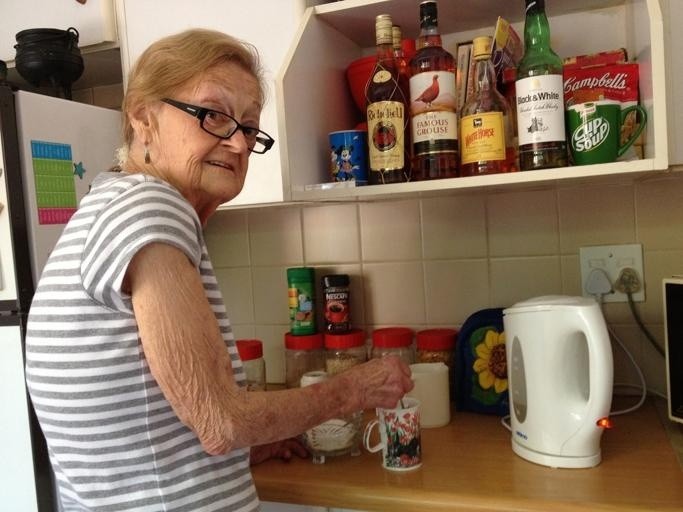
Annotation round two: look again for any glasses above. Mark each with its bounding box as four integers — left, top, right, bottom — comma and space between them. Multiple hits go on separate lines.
159, 99, 274, 154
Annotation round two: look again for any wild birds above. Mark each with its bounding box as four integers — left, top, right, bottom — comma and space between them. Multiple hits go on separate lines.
414, 73, 440, 109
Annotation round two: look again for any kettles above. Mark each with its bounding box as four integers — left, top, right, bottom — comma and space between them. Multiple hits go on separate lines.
500, 291, 617, 459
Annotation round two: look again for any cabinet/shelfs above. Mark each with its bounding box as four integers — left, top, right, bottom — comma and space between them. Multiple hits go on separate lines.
1, 0, 668, 212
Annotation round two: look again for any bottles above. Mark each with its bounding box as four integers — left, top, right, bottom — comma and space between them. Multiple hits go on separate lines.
235, 337, 268, 395
362, 9, 411, 186
323, 329, 370, 377
282, 332, 328, 391
414, 326, 461, 368
368, 326, 413, 367
320, 272, 353, 334
407, 0, 460, 184
299, 371, 327, 389
512, 0, 570, 173
285, 264, 317, 337
456, 34, 519, 179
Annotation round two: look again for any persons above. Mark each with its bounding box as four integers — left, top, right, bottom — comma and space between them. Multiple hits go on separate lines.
23, 23, 417, 512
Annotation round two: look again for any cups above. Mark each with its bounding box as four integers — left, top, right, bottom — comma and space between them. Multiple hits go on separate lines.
400, 362, 451, 429
362, 397, 423, 473
327, 127, 370, 187
563, 99, 648, 167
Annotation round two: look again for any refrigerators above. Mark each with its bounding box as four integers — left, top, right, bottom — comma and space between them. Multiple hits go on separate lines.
0, 56, 155, 511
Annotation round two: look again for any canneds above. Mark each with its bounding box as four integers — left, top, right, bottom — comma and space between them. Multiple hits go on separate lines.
323, 274, 350, 334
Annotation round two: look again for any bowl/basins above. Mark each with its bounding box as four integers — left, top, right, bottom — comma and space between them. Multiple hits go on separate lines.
296, 410, 367, 461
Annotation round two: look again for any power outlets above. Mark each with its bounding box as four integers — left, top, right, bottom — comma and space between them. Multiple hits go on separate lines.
581, 244, 648, 308
